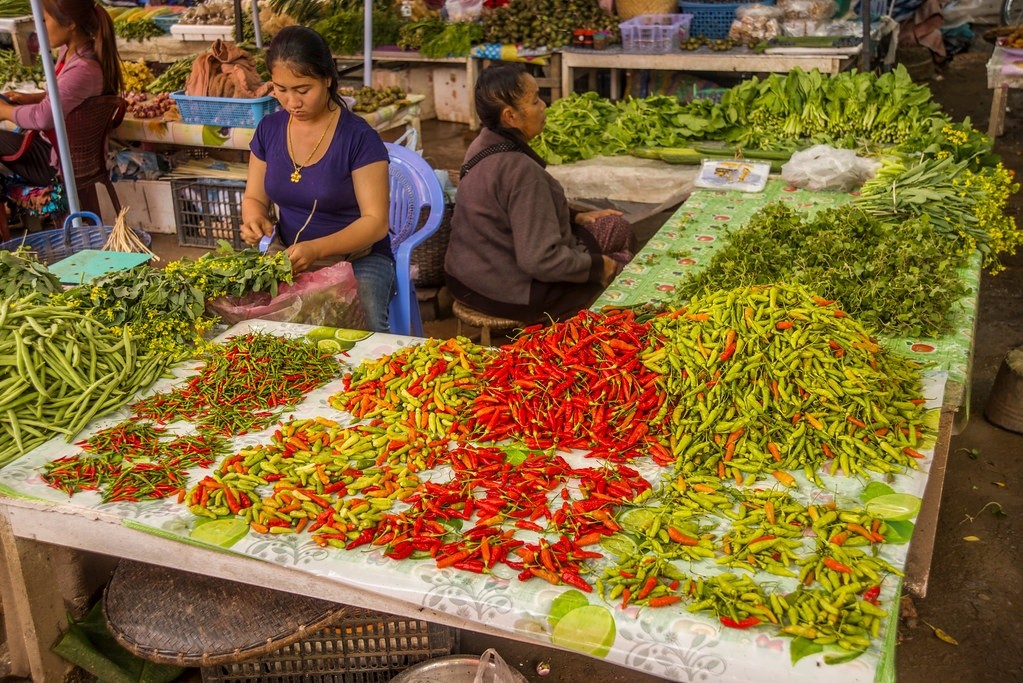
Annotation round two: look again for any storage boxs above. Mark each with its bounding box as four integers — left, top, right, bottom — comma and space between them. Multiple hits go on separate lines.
678, 0, 776, 39
94, 178, 177, 235
372, 63, 469, 123
169, 89, 280, 129
200, 607, 460, 683
170, 178, 250, 252
169, 23, 236, 42
618, 12, 696, 54
152, 12, 181, 34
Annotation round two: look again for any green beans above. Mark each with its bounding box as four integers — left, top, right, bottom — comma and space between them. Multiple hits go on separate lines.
0, 291, 192, 472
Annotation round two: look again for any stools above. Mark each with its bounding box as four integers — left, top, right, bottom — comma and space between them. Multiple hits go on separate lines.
452, 301, 526, 345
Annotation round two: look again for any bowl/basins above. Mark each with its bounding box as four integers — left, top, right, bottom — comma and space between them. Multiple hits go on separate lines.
388, 653, 529, 683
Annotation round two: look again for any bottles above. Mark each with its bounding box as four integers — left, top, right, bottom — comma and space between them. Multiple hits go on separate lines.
594, 34, 605, 49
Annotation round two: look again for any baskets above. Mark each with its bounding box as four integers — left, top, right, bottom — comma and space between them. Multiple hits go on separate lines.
618, 14, 694, 52
170, 89, 282, 129
151, 12, 181, 34
409, 192, 458, 286
678, 0, 774, 38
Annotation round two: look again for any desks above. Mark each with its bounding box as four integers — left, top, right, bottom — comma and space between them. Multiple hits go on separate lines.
560, 19, 889, 100
0, 14, 38, 67
470, 41, 596, 104
330, 51, 482, 131
0, 318, 939, 683
552, 156, 983, 599
113, 34, 215, 64
986, 37, 1023, 140
108, 93, 425, 153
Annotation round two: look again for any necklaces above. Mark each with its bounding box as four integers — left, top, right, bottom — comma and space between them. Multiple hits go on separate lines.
288, 108, 337, 184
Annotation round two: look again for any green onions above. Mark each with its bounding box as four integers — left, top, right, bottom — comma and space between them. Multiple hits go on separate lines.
256, 0, 322, 34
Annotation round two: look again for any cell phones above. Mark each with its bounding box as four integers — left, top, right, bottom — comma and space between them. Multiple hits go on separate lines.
0, 94, 18, 106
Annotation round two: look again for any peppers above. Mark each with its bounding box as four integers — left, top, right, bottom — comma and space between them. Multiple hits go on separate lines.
38, 285, 937, 654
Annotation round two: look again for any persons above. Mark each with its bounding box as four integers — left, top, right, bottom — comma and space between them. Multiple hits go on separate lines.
240, 24, 396, 333
0, 0, 129, 202
444, 65, 636, 322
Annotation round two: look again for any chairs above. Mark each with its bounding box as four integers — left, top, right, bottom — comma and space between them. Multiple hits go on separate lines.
257, 141, 444, 336
0, 94, 129, 230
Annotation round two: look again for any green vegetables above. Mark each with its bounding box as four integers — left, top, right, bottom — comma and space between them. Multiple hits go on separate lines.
146, 41, 270, 95
231, 9, 270, 43
112, 17, 164, 43
0, 238, 294, 323
673, 196, 975, 341
531, 65, 1023, 277
0, 0, 60, 89
313, 0, 484, 58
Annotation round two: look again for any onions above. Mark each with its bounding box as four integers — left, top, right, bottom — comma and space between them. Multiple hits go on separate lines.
121, 91, 177, 119
